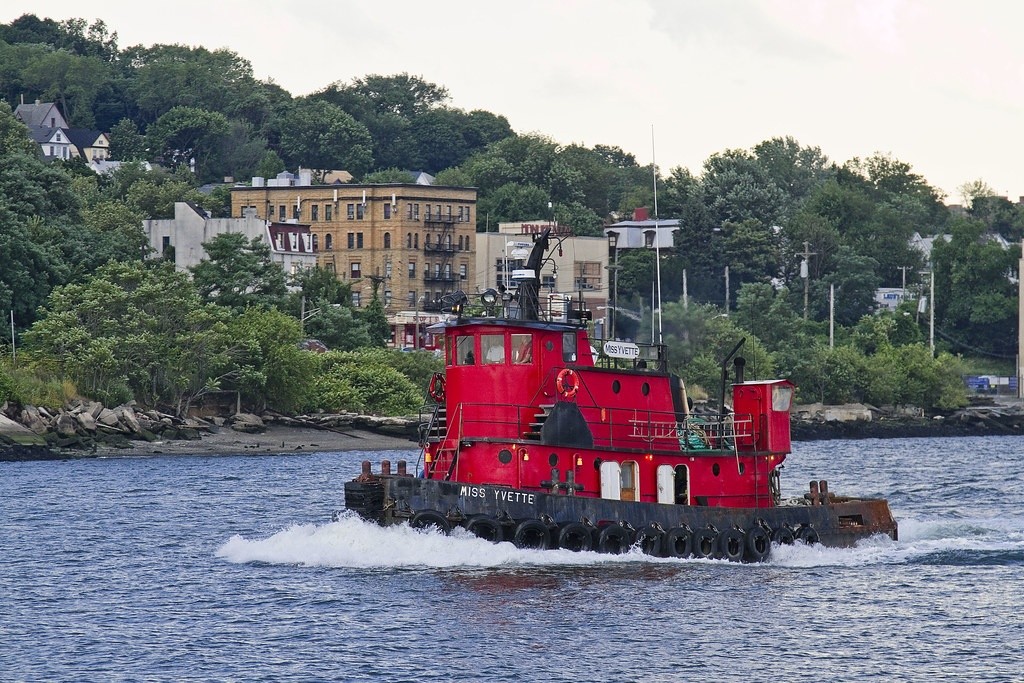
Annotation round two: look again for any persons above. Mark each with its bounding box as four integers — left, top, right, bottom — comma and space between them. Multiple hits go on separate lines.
515, 335, 531, 363
486, 336, 504, 363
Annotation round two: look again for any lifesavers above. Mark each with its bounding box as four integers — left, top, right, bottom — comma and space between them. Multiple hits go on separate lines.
429, 373, 447, 403
557, 369, 580, 398
406, 509, 820, 562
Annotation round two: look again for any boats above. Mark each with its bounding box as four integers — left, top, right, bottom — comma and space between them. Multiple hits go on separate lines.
331, 226, 899, 562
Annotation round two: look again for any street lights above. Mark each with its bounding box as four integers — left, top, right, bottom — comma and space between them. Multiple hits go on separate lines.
918, 270, 935, 359
